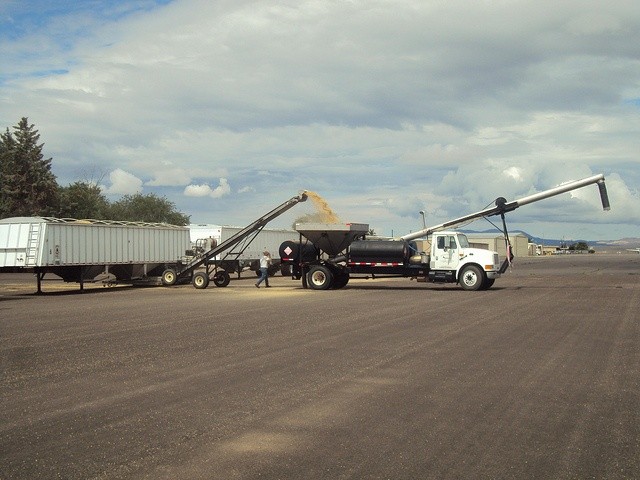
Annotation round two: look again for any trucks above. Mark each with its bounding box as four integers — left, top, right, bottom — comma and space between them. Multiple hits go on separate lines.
294, 223, 501, 291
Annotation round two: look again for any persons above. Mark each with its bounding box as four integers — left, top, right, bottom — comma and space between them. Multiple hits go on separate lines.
255, 251, 272, 288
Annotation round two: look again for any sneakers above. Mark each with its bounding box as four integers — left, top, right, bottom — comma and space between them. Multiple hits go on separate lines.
255, 284, 260, 288
265, 285, 271, 287
